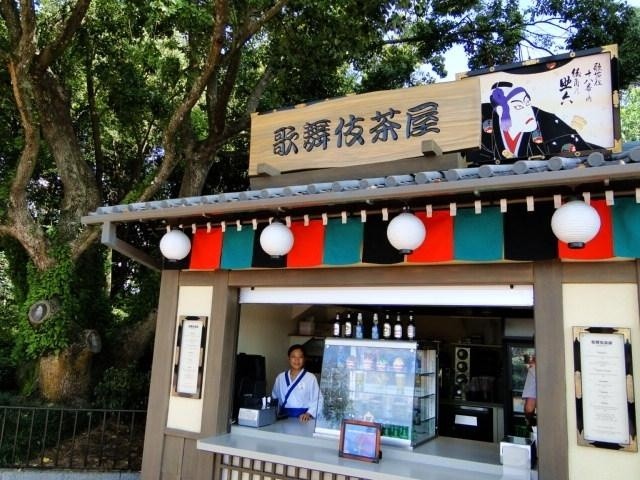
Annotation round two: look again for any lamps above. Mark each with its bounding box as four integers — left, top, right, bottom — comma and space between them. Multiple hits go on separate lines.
260, 206, 294, 261
551, 190, 601, 249
387, 204, 426, 255
159, 218, 191, 263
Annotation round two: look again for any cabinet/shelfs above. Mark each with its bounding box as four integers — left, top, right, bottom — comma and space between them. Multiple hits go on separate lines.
313, 337, 440, 451
287, 295, 505, 443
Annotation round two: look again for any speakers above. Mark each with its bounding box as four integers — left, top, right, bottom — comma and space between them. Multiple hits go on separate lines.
453, 347, 471, 403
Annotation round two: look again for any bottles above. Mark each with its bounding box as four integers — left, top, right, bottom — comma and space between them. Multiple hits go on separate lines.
332, 311, 342, 336
371, 313, 380, 339
355, 313, 364, 338
344, 311, 353, 337
383, 310, 392, 338
394, 311, 403, 339
407, 310, 416, 339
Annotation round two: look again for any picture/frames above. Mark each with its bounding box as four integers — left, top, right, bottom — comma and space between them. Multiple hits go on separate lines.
572, 325, 639, 453
338, 419, 382, 466
171, 315, 209, 400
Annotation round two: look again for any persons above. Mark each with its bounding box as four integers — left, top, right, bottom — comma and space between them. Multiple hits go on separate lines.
270, 344, 323, 422
521, 332, 536, 420
